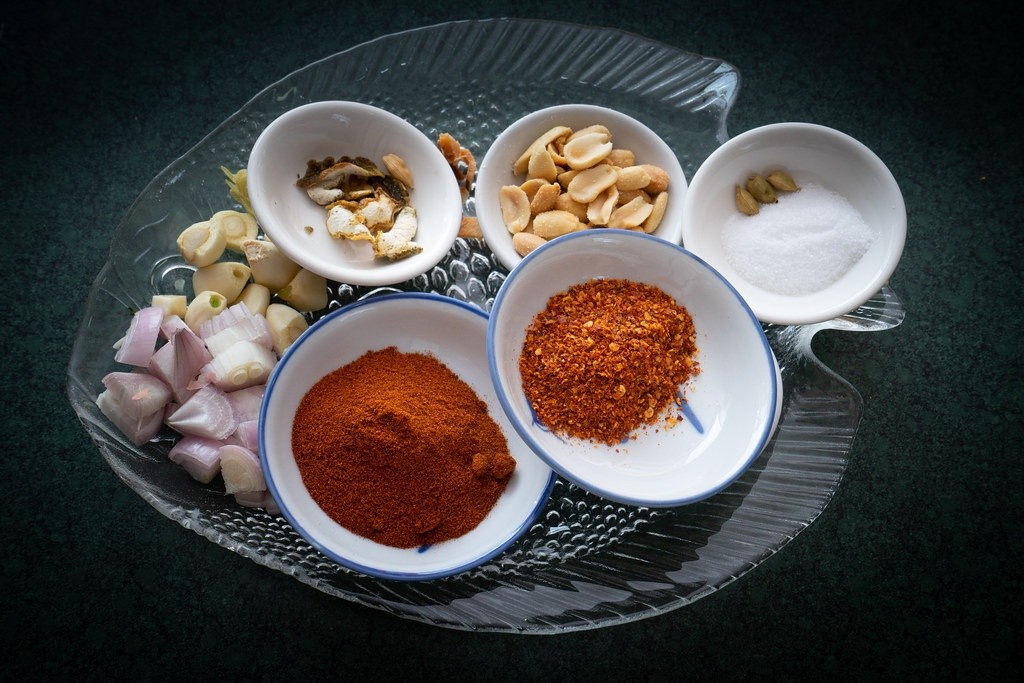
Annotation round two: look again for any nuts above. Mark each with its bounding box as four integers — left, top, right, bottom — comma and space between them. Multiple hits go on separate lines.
498, 126, 667, 256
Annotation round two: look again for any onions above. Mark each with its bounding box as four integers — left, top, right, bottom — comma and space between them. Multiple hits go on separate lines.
96, 301, 282, 517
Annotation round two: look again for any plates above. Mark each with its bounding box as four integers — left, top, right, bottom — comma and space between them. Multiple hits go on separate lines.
473, 101, 690, 273
678, 121, 911, 326
243, 98, 463, 286
486, 230, 779, 511
64, 16, 906, 638
262, 295, 553, 584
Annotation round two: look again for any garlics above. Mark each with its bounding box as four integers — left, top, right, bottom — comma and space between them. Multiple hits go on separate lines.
151, 209, 328, 360
